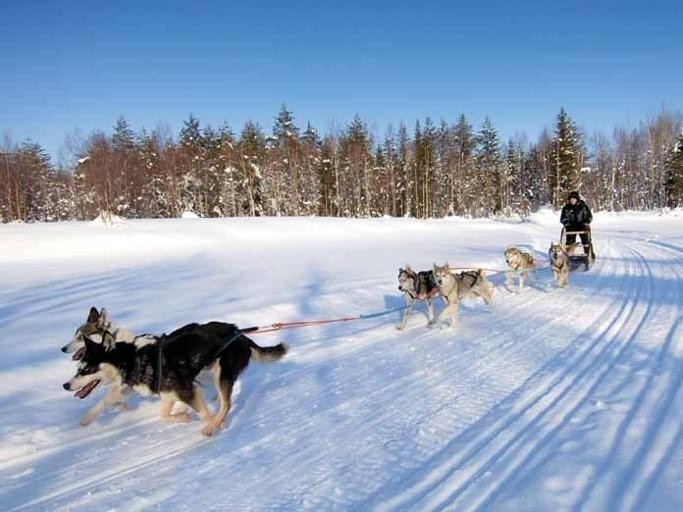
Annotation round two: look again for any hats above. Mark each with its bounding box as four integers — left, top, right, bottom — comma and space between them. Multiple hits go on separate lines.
568, 191, 580, 202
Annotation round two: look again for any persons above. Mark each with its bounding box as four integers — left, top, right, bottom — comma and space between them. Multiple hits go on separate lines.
559, 191, 596, 263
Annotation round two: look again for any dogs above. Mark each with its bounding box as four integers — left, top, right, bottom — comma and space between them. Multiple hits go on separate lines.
548, 241, 571, 288
61, 305, 289, 437
427, 261, 497, 331
503, 245, 538, 294
393, 263, 441, 331
